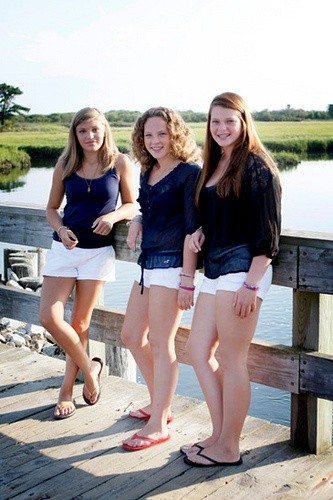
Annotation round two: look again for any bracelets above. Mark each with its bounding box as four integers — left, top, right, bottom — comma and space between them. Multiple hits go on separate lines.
178, 273, 195, 278
178, 284, 195, 291
57, 226, 67, 237
243, 282, 259, 291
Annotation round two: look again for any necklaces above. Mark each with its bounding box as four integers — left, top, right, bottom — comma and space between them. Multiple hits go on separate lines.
82, 163, 99, 192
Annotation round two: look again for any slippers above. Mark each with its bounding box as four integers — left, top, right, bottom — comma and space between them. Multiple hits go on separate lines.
122, 432, 171, 451
53, 398, 77, 420
183, 449, 243, 467
82, 357, 103, 405
128, 406, 174, 422
180, 442, 204, 455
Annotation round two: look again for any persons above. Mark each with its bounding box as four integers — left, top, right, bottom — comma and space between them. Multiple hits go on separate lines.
181, 92, 282, 468
39, 107, 142, 418
120, 106, 203, 450
